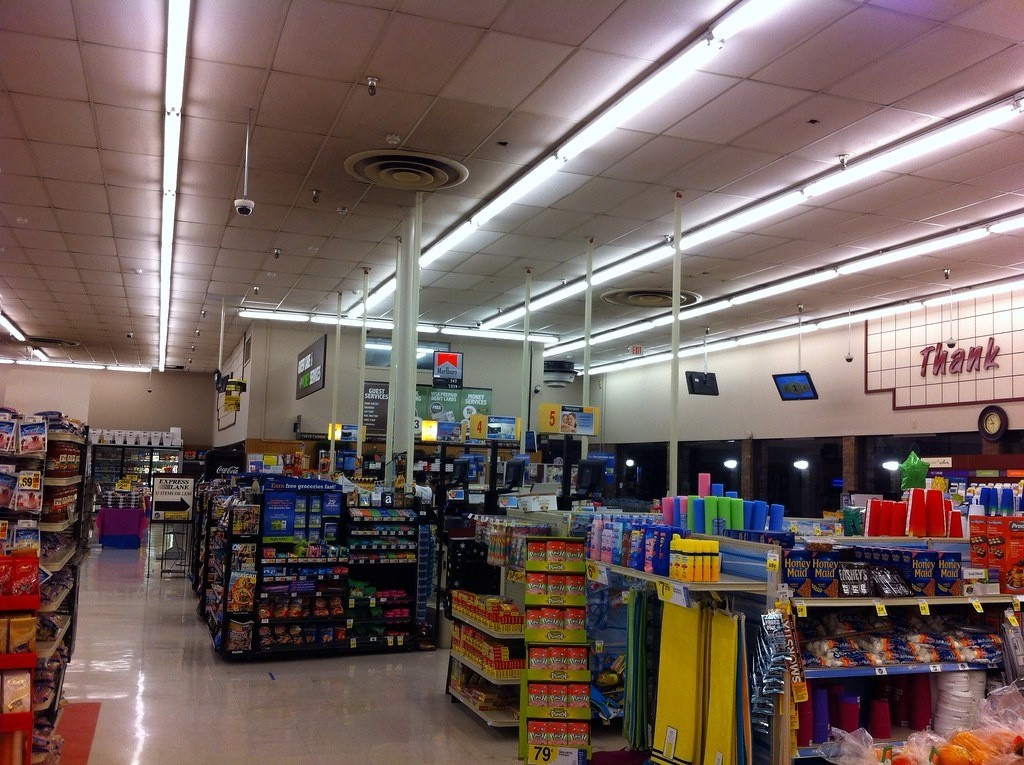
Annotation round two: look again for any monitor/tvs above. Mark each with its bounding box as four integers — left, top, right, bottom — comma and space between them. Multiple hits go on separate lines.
571, 460, 606, 499
685, 371, 719, 396
525, 431, 537, 453
772, 371, 819, 401
450, 458, 470, 488
498, 459, 526, 493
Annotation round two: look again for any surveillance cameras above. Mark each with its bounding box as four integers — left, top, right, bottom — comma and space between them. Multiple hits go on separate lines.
126, 333, 133, 338
235, 199, 255, 215
148, 389, 152, 393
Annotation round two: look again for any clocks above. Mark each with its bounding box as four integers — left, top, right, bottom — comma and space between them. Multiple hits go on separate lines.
978, 405, 1008, 441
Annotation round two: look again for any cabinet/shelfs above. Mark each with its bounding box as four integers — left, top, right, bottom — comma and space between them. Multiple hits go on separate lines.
519, 536, 592, 765
191, 471, 438, 664
91, 444, 182, 513
0, 405, 90, 765
96, 495, 151, 548
445, 492, 569, 728
586, 519, 1024, 765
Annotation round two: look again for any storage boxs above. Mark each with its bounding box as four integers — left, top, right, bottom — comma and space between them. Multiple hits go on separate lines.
450, 586, 526, 715
91, 427, 185, 445
